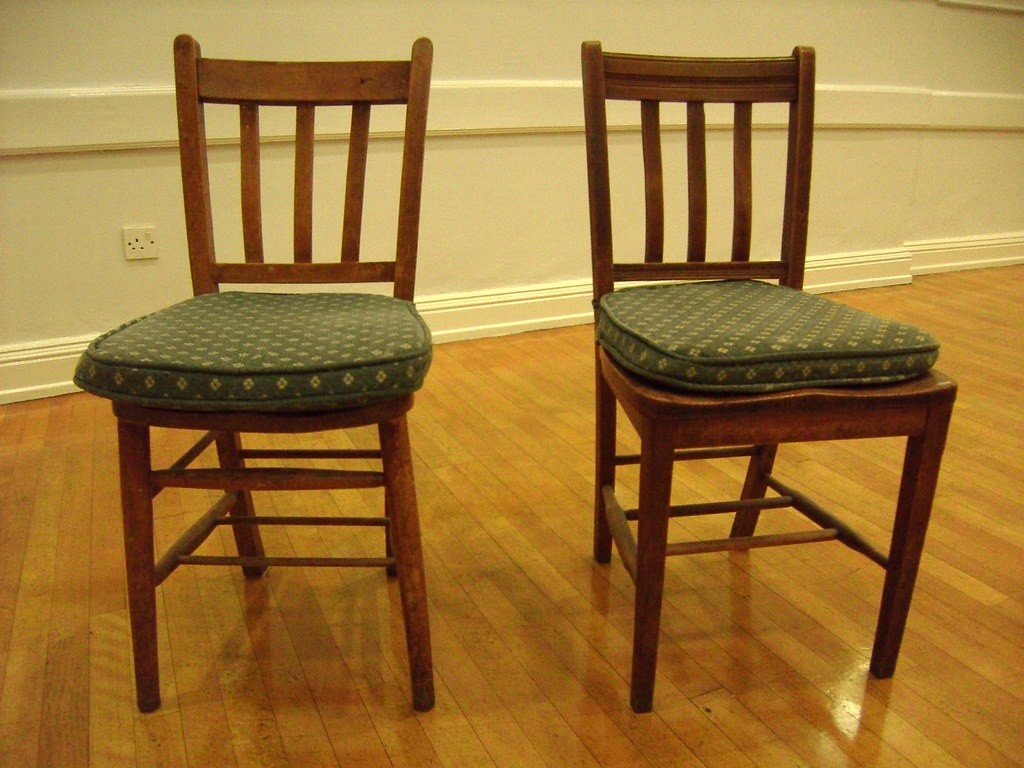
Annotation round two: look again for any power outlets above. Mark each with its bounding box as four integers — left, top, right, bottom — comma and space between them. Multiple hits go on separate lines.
121, 226, 160, 260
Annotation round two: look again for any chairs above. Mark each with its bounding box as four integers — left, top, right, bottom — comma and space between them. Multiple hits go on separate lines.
580, 40, 959, 712
70, 31, 438, 718
